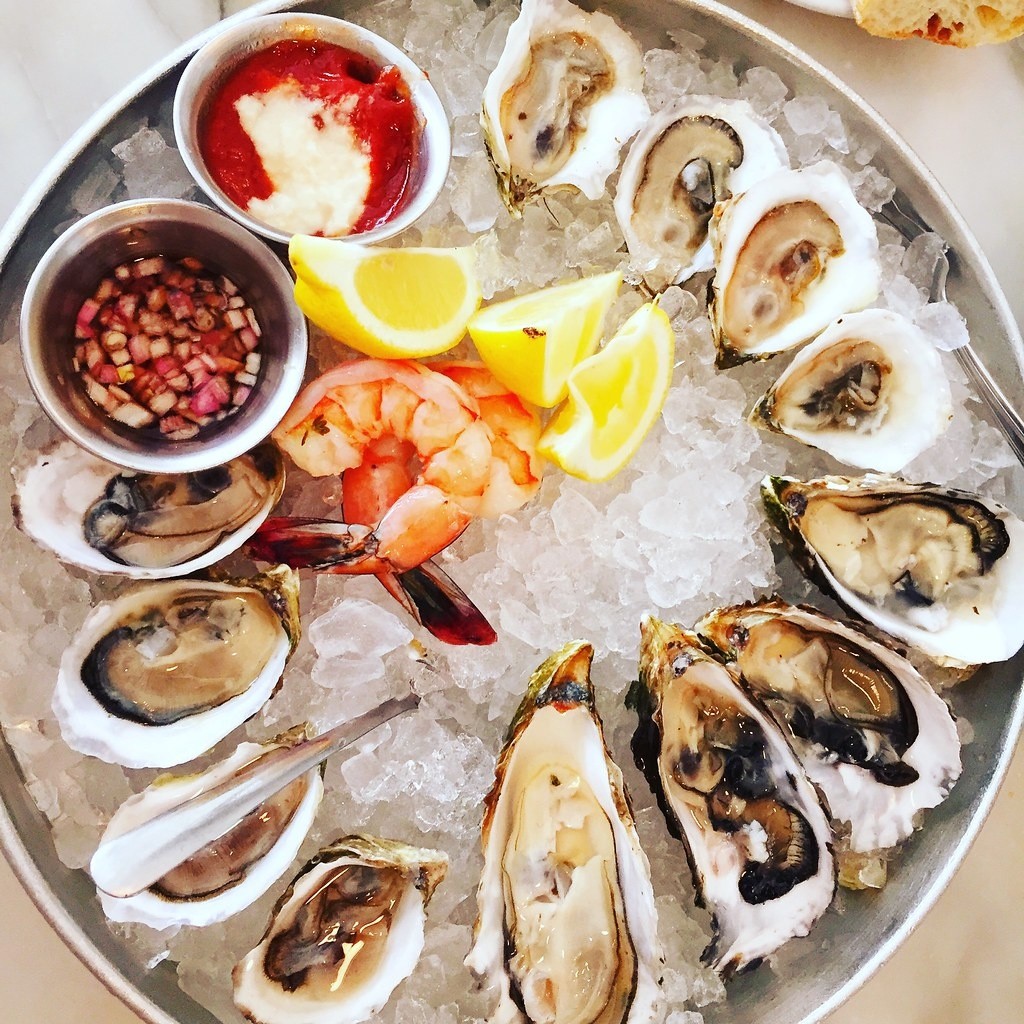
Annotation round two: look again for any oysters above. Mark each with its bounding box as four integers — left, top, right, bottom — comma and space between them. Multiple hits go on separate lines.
464, 4, 1023, 1023
8, 425, 451, 1024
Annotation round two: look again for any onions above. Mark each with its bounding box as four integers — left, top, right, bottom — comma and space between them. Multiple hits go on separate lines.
72, 255, 262, 440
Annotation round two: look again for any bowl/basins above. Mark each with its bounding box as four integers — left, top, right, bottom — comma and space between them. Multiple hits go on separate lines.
18, 197, 308, 476
171, 11, 453, 247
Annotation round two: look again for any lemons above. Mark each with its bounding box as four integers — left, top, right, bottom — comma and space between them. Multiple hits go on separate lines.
466, 269, 623, 407
286, 231, 484, 359
534, 300, 677, 484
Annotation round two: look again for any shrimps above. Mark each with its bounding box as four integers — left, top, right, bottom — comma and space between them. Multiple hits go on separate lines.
237, 355, 536, 648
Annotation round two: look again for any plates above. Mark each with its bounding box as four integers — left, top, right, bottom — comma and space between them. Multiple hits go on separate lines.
0, 0, 1024, 1024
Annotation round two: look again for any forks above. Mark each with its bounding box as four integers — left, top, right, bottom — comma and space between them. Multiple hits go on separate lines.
871, 200, 1024, 469
